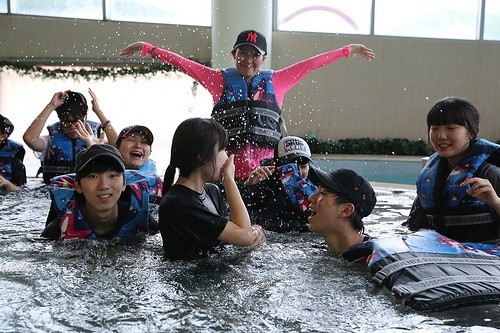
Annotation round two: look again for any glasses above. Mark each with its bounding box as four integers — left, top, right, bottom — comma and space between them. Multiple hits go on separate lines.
59, 115, 85, 128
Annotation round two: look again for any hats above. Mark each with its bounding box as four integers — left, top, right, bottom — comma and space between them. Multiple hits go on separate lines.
55, 92, 88, 110
234, 30, 268, 56
0, 114, 14, 137
309, 165, 377, 218
118, 125, 154, 145
75, 144, 126, 172
274, 134, 315, 163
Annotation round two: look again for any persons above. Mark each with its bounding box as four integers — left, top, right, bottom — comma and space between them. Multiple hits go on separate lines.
158, 117, 265, 262
0, 114, 27, 192
72, 87, 162, 210
23, 91, 106, 181
402, 97, 500, 245
245, 137, 332, 189
41, 144, 160, 244
119, 30, 375, 180
308, 170, 377, 261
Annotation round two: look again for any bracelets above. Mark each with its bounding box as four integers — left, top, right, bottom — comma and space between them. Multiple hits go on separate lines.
101, 120, 110, 131
